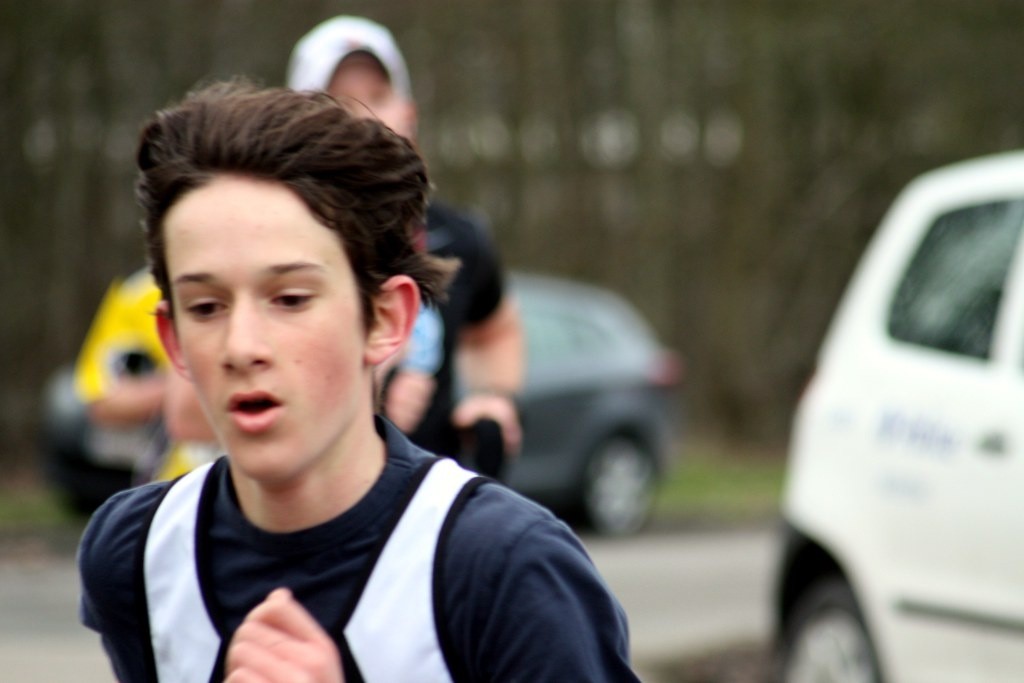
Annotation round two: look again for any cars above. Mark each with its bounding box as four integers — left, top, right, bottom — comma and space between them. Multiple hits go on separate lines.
31, 273, 687, 544
774, 142, 1024, 683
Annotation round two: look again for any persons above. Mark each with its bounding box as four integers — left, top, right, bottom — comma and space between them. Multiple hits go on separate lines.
78, 15, 523, 483
75, 81, 643, 683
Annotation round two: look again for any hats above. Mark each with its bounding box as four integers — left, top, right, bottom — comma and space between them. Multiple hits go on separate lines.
286, 14, 410, 104
